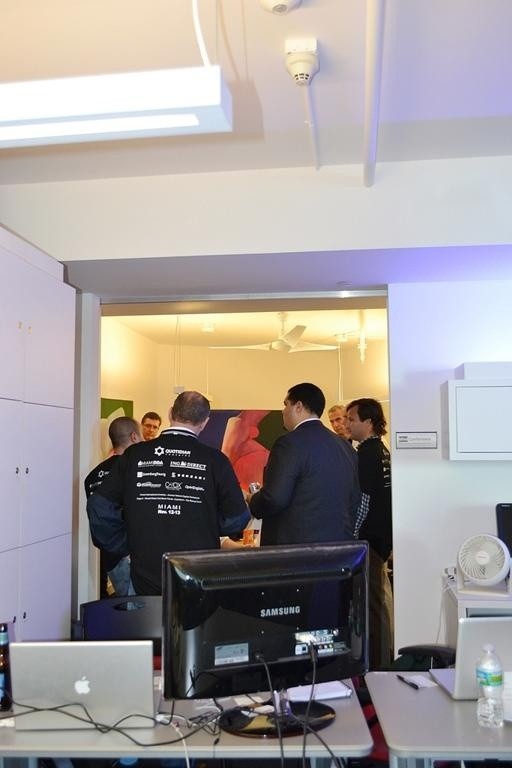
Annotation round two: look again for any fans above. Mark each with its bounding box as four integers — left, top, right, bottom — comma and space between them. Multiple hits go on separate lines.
453, 532, 510, 598
203, 307, 337, 355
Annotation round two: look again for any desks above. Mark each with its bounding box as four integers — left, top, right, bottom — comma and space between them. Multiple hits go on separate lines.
0, 678, 375, 767
363, 668, 512, 767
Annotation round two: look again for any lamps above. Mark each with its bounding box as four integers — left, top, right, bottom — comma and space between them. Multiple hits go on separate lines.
0, 1, 232, 149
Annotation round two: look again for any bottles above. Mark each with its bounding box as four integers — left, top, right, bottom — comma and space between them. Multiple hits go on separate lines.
0, 623, 12, 712
474, 643, 505, 731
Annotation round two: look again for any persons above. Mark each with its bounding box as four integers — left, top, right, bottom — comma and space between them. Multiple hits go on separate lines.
218, 528, 253, 551
85, 389, 252, 611
346, 397, 392, 669
140, 411, 161, 440
327, 404, 360, 454
246, 381, 362, 546
83, 416, 146, 610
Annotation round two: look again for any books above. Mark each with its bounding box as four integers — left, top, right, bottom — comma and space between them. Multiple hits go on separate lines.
286, 677, 353, 703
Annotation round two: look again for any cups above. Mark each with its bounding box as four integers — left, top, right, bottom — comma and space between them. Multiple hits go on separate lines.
248, 482, 262, 492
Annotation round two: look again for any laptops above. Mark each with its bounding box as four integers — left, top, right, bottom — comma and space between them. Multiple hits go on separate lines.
9, 640, 162, 730
428, 616, 512, 700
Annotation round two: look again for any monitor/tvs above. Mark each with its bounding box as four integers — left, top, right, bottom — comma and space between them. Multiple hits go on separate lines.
163, 540, 369, 737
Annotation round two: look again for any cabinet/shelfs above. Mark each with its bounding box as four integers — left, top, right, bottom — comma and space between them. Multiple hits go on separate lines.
0, 229, 78, 409
440, 577, 511, 647
439, 361, 512, 462
1, 532, 75, 647
1, 398, 71, 550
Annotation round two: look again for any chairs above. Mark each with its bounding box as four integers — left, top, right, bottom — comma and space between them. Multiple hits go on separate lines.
79, 594, 162, 672
454, 615, 511, 669
